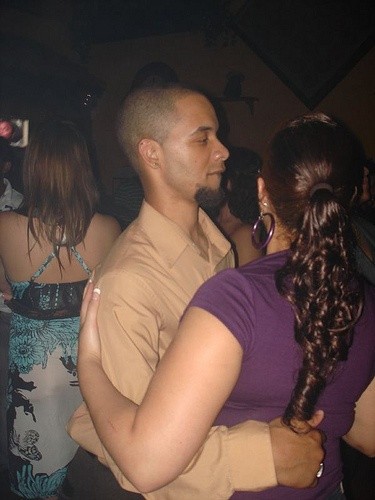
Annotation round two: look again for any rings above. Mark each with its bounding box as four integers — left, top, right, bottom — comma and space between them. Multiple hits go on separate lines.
317, 463, 324, 479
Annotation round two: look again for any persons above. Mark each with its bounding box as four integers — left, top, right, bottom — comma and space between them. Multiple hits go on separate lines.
76, 115, 375, 499
346, 153, 375, 286
0, 113, 123, 500
0, 136, 30, 216
61, 84, 327, 500
195, 146, 269, 268
